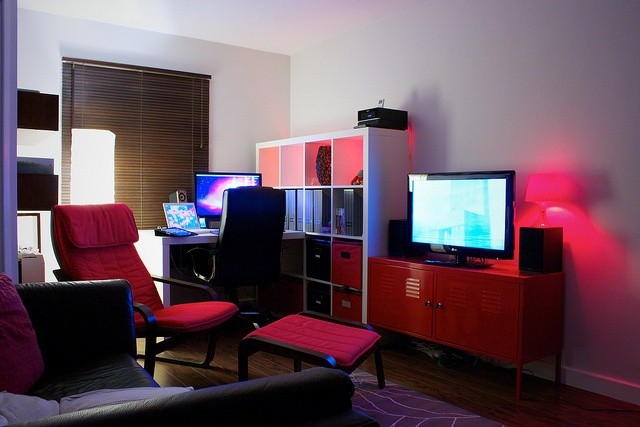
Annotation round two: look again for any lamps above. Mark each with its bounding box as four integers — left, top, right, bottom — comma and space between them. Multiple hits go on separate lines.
70, 128, 116, 205
524, 168, 577, 229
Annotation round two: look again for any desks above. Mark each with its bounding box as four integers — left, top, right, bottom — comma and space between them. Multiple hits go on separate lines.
153, 228, 305, 339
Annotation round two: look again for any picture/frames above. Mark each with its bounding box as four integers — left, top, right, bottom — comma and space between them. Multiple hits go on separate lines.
18, 212, 42, 257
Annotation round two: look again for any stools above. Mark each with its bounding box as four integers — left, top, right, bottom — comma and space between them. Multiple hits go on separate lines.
238, 311, 386, 392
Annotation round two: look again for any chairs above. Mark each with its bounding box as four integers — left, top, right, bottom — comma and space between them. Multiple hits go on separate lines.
52, 202, 238, 380
187, 186, 287, 324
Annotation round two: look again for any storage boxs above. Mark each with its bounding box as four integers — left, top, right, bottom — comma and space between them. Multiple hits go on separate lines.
332, 239, 362, 291
305, 238, 330, 281
306, 284, 331, 316
334, 287, 362, 323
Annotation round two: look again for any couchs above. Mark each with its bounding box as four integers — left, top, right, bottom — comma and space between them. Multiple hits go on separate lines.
1, 277, 355, 426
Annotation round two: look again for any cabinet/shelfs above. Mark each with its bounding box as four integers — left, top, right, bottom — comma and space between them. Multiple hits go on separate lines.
254, 126, 410, 327
20, 253, 46, 283
369, 256, 566, 400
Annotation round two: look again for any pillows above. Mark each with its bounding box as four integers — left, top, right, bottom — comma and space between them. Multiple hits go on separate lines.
59, 386, 194, 413
0, 272, 46, 392
0, 391, 60, 426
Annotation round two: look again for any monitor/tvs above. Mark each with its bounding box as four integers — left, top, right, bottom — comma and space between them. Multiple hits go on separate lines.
406, 170, 515, 269
195, 172, 262, 228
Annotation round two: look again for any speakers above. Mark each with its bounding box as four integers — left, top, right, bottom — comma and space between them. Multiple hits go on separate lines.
388, 218, 427, 258
518, 226, 563, 274
169, 189, 188, 203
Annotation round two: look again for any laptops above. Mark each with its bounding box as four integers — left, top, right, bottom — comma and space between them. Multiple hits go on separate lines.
162, 202, 220, 234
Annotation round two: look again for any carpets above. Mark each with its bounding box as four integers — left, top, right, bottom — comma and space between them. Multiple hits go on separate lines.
350, 368, 508, 426
